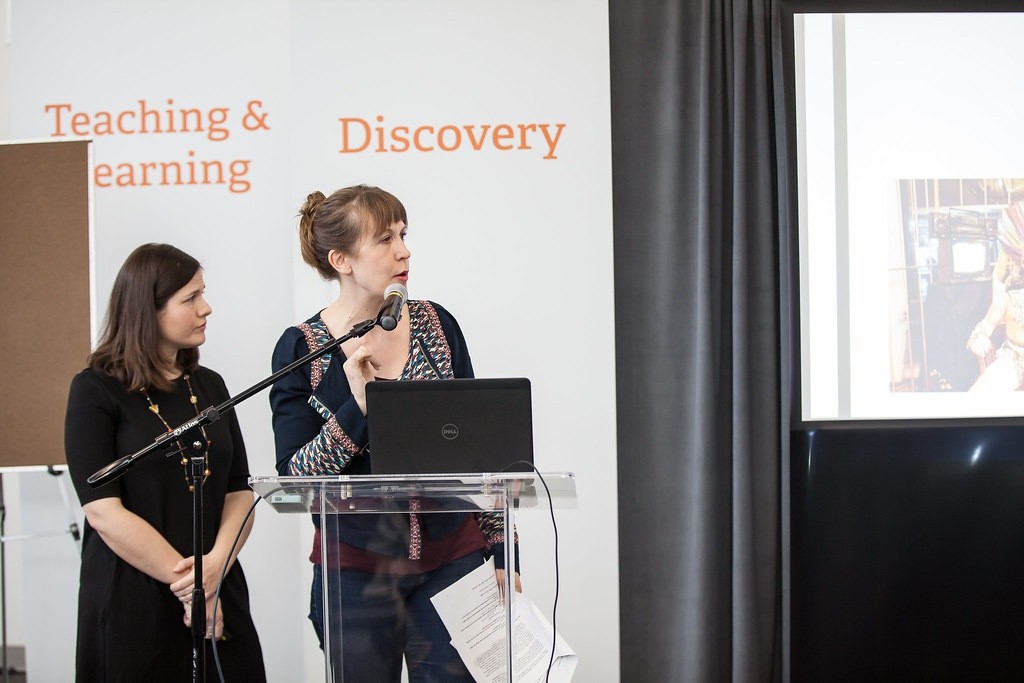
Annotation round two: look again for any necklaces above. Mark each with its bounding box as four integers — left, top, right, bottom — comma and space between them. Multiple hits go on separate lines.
140, 373, 210, 494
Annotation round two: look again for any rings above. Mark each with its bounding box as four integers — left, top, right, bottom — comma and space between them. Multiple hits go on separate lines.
207, 626, 212, 628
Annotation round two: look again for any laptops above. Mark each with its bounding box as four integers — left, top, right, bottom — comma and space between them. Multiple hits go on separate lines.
365, 377, 535, 486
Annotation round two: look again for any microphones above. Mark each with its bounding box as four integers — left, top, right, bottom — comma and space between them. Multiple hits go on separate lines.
379, 283, 408, 330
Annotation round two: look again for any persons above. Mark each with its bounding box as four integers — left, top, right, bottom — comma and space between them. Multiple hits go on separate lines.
63, 243, 267, 683
270, 186, 522, 683
